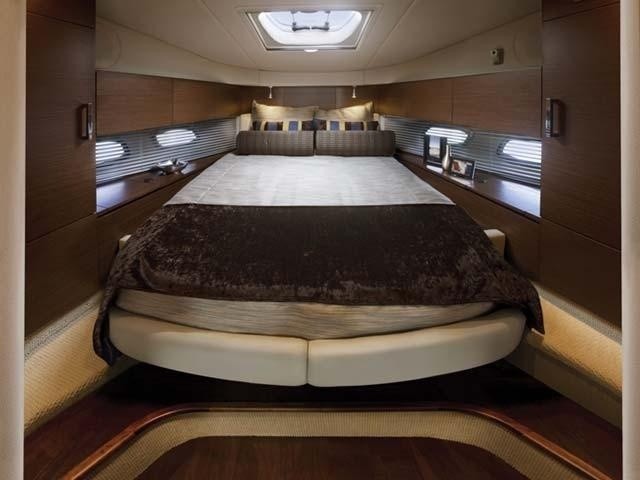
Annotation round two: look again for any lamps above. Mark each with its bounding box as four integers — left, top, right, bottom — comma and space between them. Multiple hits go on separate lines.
79, 103, 93, 141
543, 99, 562, 139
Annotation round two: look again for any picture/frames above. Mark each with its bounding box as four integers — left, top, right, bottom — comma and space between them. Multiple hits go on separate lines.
447, 155, 476, 179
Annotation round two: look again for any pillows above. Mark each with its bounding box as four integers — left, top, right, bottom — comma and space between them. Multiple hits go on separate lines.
236, 99, 396, 157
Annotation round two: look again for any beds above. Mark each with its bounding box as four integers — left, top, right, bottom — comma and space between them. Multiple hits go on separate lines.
97, 113, 531, 388
